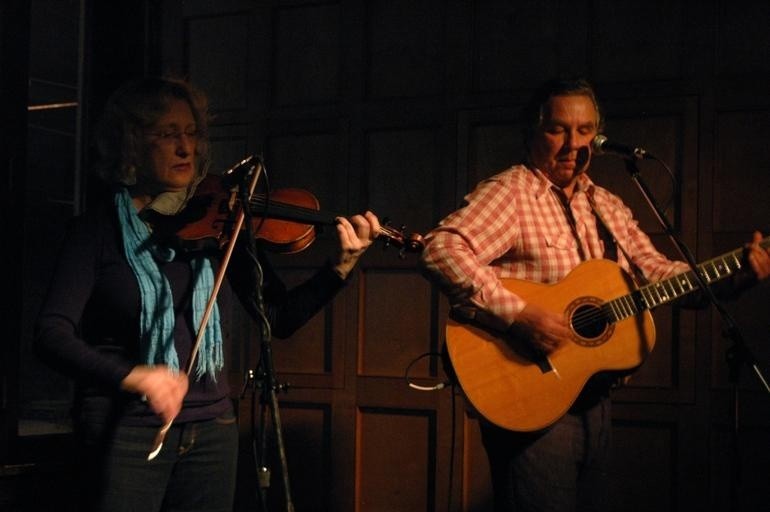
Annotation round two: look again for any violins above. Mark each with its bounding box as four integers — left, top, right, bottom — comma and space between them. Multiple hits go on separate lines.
171, 172, 428, 256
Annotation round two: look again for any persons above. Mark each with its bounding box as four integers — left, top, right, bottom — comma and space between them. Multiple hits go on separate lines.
34, 75, 381, 511
417, 78, 770, 512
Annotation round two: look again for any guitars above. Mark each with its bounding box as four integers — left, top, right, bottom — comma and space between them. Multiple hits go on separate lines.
443, 234, 769, 431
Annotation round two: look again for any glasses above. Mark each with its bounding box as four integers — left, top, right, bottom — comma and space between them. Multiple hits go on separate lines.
142, 128, 200, 144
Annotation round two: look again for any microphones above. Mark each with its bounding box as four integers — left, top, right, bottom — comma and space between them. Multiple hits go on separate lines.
590, 134, 647, 159
216, 164, 250, 193
